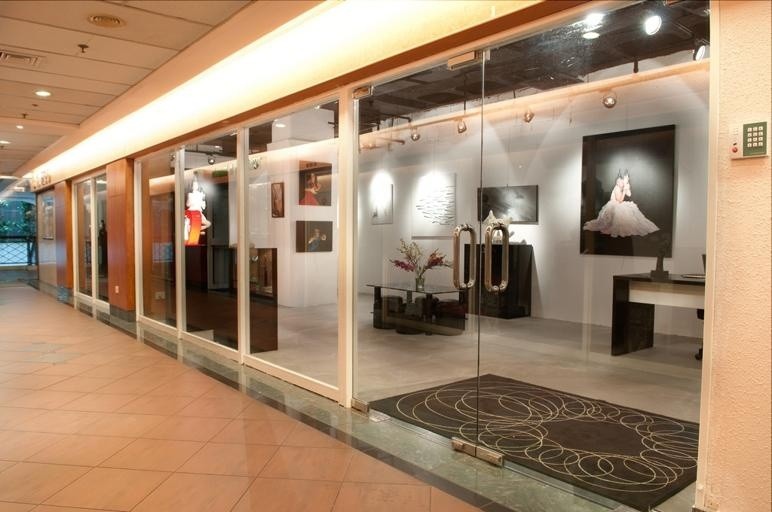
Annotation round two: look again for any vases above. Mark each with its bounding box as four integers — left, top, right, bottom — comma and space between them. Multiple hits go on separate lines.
415, 274, 425, 290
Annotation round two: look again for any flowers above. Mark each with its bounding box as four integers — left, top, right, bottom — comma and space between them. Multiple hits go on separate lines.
387, 237, 456, 277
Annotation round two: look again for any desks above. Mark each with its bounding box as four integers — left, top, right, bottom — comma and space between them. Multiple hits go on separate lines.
611, 273, 704, 356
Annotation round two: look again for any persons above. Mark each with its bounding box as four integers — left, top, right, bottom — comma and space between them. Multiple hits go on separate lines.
300, 172, 321, 206
308, 227, 327, 251
584, 166, 660, 237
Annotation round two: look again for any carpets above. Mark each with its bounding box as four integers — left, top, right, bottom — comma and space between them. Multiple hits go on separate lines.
366, 372, 701, 511
